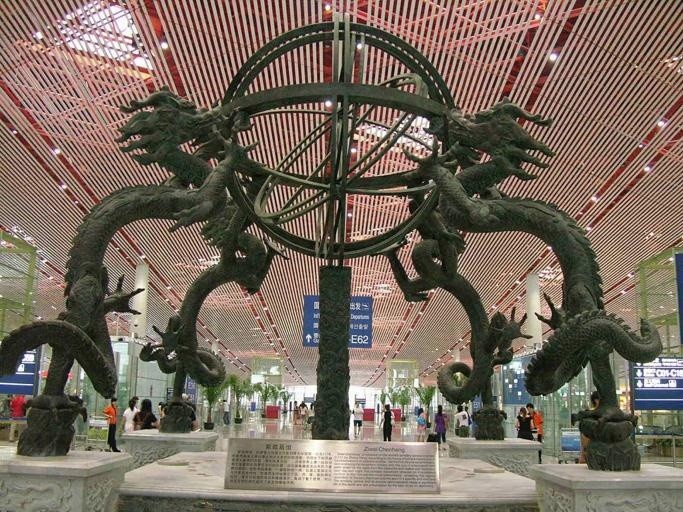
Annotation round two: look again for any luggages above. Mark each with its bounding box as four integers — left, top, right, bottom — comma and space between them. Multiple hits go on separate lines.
426, 433, 439, 443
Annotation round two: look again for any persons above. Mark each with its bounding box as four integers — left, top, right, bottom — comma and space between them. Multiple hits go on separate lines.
157, 393, 198, 430
3, 393, 14, 417
292, 400, 315, 432
221, 399, 231, 425
8, 394, 26, 442
132, 398, 159, 431
577, 389, 600, 464
351, 401, 364, 438
118, 399, 140, 432
525, 402, 543, 464
514, 406, 535, 440
101, 396, 120, 452
131, 396, 139, 409
417, 404, 469, 450
377, 403, 395, 440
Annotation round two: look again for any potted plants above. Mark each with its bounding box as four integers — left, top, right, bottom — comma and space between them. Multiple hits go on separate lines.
399, 374, 467, 436
200, 374, 291, 430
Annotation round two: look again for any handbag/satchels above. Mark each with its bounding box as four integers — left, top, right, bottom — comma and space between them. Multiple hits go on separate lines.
390, 416, 396, 427
468, 416, 473, 426
